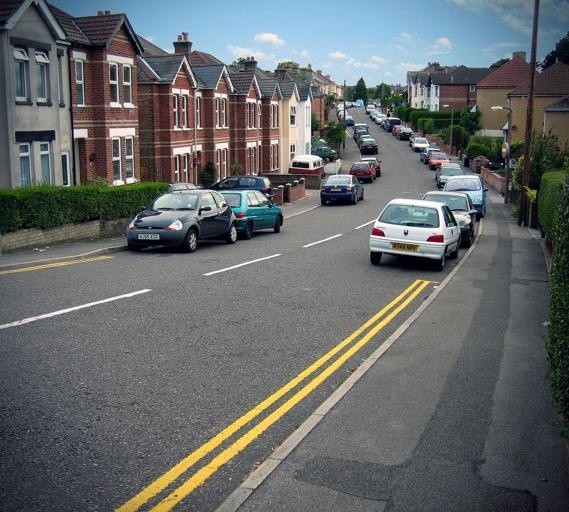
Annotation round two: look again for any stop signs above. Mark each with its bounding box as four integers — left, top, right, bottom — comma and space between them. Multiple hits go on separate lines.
501, 141, 510, 159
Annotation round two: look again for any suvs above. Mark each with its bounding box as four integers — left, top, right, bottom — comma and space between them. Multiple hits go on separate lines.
204, 176, 274, 203
360, 157, 382, 178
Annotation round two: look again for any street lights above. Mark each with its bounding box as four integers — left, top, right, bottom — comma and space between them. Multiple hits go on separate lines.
490, 105, 512, 205
442, 105, 454, 157
398, 94, 407, 127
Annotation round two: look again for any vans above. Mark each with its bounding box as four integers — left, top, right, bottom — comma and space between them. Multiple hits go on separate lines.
288, 154, 326, 179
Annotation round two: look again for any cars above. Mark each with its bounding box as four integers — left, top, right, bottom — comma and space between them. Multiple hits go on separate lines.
350, 162, 377, 185
168, 182, 200, 193
220, 188, 283, 240
421, 190, 480, 248
443, 172, 489, 218
336, 98, 423, 154
368, 198, 465, 271
429, 151, 450, 170
320, 174, 365, 206
412, 137, 430, 153
126, 189, 238, 252
421, 147, 441, 165
436, 163, 463, 188
